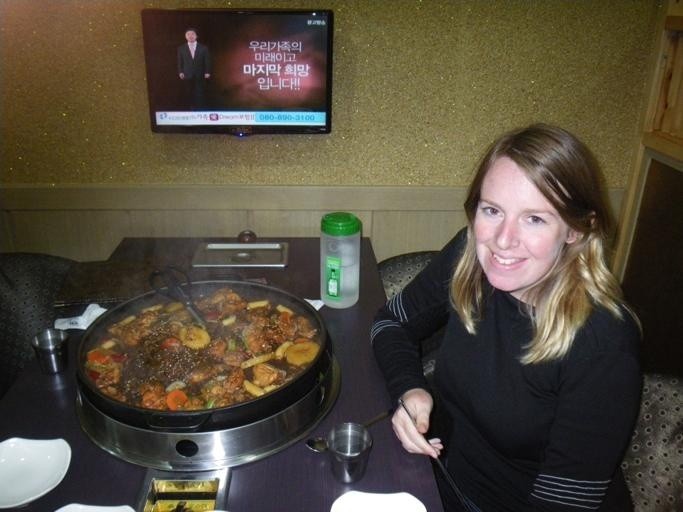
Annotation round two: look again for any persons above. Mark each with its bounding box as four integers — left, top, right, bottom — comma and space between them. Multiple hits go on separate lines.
367, 123, 645, 512
176, 28, 213, 111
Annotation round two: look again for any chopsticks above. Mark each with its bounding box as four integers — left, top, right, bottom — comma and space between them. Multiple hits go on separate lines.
52, 296, 131, 309
398, 397, 484, 512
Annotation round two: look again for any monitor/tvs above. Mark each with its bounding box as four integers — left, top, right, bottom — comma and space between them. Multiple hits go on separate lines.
142, 8, 334, 137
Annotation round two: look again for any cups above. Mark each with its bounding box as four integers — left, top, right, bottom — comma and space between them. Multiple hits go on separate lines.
30, 329, 70, 376
325, 421, 375, 484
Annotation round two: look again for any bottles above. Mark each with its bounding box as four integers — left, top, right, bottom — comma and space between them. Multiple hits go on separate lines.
318, 211, 363, 310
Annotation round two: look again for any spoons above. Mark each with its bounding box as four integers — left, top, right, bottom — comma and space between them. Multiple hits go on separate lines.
305, 407, 394, 456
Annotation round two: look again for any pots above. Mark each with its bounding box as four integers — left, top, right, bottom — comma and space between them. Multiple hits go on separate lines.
73, 275, 345, 434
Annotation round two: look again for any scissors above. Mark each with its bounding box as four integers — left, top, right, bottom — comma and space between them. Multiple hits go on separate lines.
148, 263, 209, 330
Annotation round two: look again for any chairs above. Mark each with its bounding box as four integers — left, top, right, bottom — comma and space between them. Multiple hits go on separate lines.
621, 367, 683, 512
0, 250, 105, 398
377, 250, 447, 385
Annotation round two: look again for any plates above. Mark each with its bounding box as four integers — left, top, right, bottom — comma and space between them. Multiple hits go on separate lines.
0, 436, 73, 509
329, 490, 428, 512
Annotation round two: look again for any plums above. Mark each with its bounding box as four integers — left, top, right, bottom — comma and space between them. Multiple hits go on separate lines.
239, 230, 257, 243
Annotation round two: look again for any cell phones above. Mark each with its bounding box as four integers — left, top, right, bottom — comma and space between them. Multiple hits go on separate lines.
54, 316, 87, 331
